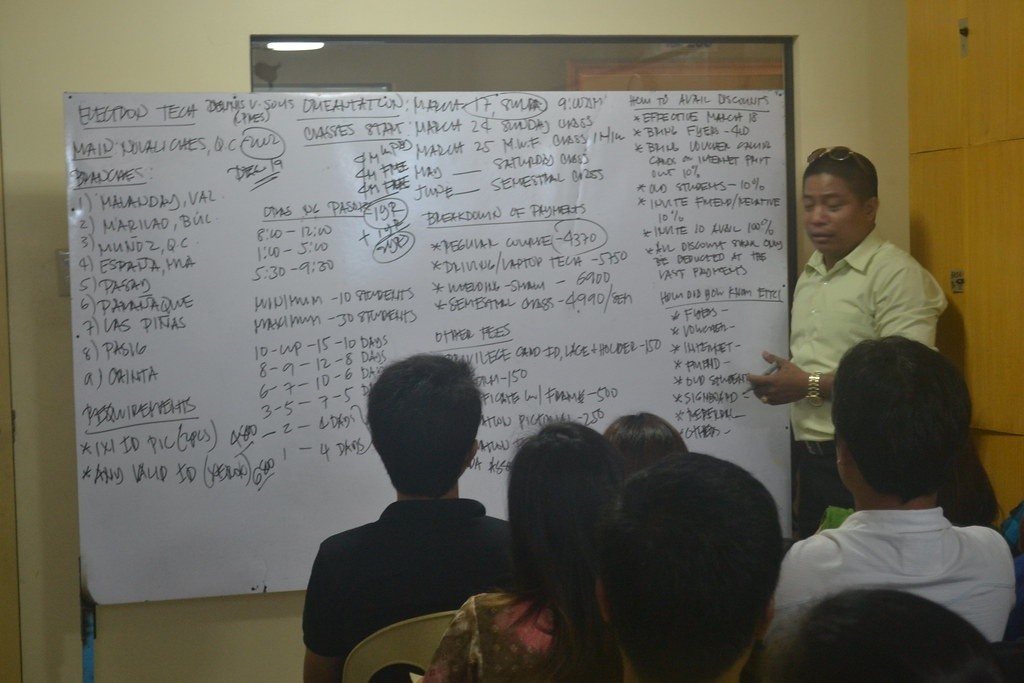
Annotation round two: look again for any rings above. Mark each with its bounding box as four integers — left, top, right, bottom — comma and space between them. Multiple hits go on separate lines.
761, 395, 768, 403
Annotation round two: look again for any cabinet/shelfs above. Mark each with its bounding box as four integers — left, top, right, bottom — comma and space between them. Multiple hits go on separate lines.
907, 0, 1024, 539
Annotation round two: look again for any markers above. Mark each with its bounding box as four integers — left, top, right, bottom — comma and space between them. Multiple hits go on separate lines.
741, 360, 781, 397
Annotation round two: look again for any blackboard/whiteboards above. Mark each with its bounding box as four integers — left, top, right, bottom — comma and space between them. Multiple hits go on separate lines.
65, 89, 793, 603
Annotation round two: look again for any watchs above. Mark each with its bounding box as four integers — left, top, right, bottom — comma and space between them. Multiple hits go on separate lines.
805, 370, 824, 407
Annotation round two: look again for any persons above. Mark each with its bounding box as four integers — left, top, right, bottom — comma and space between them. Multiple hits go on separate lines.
736, 337, 1018, 682
745, 145, 948, 543
297, 355, 517, 682
417, 411, 1023, 682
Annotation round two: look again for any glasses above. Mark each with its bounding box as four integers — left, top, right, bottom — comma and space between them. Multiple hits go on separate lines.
807, 146, 871, 176
